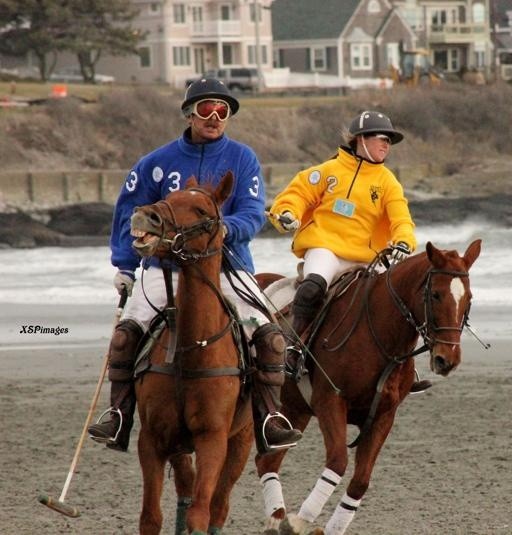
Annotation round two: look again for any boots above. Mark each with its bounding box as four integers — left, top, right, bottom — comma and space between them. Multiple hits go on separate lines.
284, 315, 311, 373
86, 367, 136, 452
410, 379, 433, 392
248, 356, 303, 456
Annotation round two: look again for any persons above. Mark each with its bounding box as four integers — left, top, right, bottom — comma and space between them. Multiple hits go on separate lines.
85, 78, 303, 458
268, 109, 434, 395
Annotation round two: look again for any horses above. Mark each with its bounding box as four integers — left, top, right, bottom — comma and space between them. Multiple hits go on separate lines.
253, 237, 483, 535
130, 172, 255, 535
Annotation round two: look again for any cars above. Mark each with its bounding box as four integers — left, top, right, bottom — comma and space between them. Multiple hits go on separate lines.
185, 67, 267, 93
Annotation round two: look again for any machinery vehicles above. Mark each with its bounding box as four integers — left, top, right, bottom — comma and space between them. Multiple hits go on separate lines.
390, 50, 441, 88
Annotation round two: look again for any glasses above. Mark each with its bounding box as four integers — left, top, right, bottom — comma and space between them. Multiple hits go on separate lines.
186, 98, 233, 122
373, 133, 392, 146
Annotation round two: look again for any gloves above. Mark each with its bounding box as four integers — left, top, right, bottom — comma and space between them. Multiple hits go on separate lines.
114, 268, 136, 297
390, 242, 412, 261
281, 211, 302, 231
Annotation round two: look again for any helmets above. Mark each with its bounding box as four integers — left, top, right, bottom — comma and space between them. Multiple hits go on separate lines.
346, 110, 404, 146
181, 78, 240, 118
